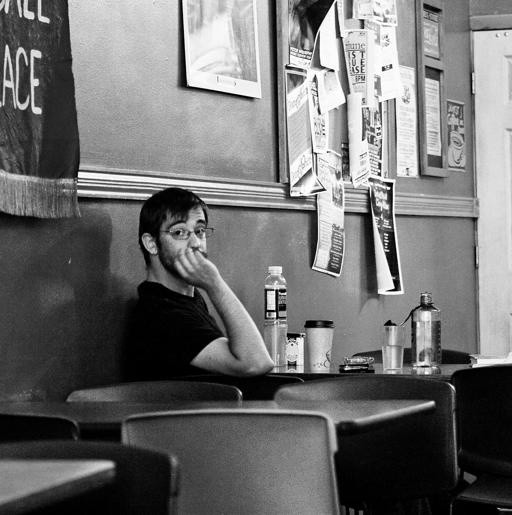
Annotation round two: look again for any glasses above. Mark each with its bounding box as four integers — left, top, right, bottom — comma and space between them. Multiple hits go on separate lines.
154, 225, 216, 240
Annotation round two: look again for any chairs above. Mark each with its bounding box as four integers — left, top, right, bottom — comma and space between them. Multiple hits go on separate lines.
1, 347, 511, 514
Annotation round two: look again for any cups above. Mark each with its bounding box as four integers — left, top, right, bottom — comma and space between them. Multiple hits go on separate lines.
380, 325, 404, 370
450, 131, 463, 162
304, 320, 335, 372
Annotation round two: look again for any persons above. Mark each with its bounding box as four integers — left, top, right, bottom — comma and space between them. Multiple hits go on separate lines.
121, 187, 274, 382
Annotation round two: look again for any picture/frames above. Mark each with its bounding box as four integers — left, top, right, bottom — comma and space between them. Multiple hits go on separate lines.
415, 0, 448, 177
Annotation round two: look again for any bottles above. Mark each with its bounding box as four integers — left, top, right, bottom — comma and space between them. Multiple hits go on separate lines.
287, 333, 304, 365
410, 293, 441, 368
264, 265, 287, 366
